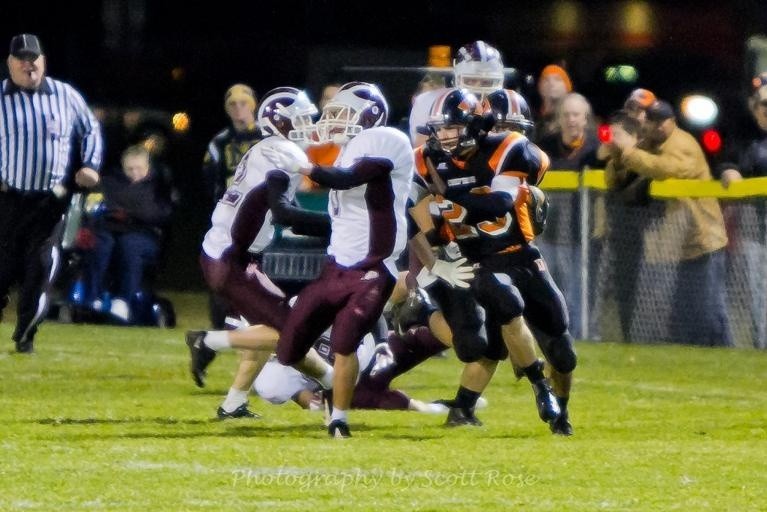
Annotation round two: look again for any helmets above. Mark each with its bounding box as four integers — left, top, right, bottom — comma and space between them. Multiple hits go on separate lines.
428, 40, 535, 158
257, 86, 319, 141
315, 81, 390, 145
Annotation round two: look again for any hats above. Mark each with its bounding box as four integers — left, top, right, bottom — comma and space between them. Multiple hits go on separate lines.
538, 64, 572, 93
224, 84, 257, 111
749, 71, 767, 114
623, 88, 656, 113
646, 99, 674, 122
10, 34, 42, 57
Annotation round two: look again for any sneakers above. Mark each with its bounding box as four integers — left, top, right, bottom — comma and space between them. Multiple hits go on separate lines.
512, 359, 573, 436
16, 339, 32, 352
394, 288, 432, 340
184, 330, 217, 388
434, 394, 487, 429
328, 418, 351, 438
216, 401, 263, 421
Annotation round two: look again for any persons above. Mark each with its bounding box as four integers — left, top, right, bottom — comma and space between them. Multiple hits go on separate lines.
0, 33, 106, 356
183, 84, 329, 421
394, 85, 565, 421
258, 79, 416, 442
225, 269, 489, 413
441, 89, 576, 433
86, 141, 173, 321
198, 41, 763, 347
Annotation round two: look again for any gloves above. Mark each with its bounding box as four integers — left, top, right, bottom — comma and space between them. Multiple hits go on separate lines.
430, 257, 476, 289
260, 145, 302, 174
